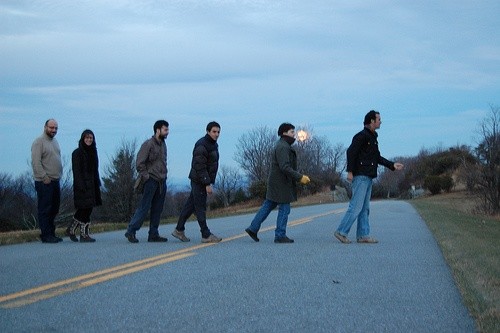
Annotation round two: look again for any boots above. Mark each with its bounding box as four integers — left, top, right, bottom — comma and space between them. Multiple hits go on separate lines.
79, 221, 96, 243
64, 217, 80, 242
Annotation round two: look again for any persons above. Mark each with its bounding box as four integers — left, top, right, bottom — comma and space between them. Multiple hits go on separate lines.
245, 123, 310, 243
125, 120, 169, 243
172, 122, 222, 242
64, 130, 102, 242
334, 111, 404, 243
32, 119, 62, 243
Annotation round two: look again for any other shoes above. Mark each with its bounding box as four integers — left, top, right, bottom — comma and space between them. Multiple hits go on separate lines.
357, 236, 377, 243
54, 236, 62, 241
202, 233, 222, 242
245, 227, 260, 242
333, 231, 352, 243
42, 237, 59, 243
147, 232, 168, 242
125, 231, 139, 243
171, 228, 190, 242
274, 236, 294, 243
